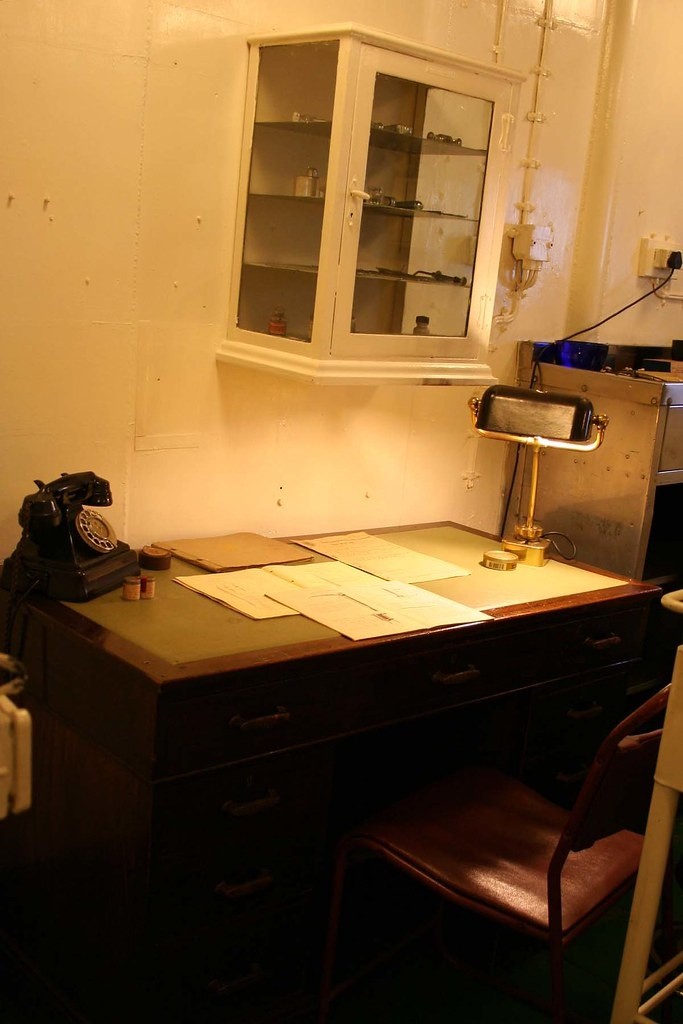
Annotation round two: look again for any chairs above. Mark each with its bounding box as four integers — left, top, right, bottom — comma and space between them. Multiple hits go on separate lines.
318, 683, 676, 1024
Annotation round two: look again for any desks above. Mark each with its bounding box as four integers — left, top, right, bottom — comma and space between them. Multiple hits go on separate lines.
0, 522, 663, 1024
498, 340, 683, 583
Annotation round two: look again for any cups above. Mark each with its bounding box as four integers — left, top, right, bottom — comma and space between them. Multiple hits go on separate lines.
294, 175, 320, 198
291, 110, 313, 122
383, 191, 396, 206
366, 182, 382, 204
534, 340, 556, 363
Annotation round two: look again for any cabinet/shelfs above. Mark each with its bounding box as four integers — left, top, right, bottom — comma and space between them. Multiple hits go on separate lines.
217, 30, 529, 387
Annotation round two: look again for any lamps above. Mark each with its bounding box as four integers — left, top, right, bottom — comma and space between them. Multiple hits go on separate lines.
469, 384, 611, 566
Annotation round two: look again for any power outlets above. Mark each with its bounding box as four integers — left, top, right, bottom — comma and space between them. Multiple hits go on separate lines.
639, 237, 678, 281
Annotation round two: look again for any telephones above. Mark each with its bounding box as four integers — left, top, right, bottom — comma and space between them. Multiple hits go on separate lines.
12, 470, 140, 605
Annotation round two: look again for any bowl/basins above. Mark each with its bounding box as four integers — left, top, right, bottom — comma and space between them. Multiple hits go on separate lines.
555, 338, 609, 371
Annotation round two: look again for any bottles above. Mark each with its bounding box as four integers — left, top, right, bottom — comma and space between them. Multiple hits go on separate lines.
397, 201, 425, 210
413, 313, 429, 336
123, 574, 155, 601
268, 305, 287, 335
